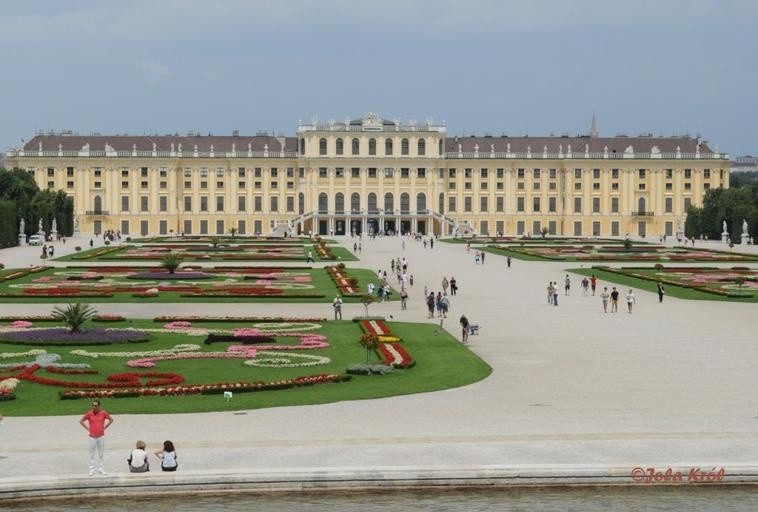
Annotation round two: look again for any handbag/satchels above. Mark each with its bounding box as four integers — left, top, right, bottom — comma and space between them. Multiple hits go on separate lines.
128, 457, 147, 465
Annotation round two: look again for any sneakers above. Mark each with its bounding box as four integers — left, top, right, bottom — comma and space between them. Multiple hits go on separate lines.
89, 468, 107, 475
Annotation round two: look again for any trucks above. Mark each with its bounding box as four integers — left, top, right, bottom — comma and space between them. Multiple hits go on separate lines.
29, 235, 45, 245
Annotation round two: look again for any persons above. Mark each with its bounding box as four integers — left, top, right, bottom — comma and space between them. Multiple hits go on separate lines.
660, 234, 695, 247
480, 249, 485, 264
79, 399, 113, 476
284, 230, 287, 238
39, 229, 122, 260
305, 229, 470, 342
474, 250, 479, 263
289, 229, 292, 238
548, 273, 666, 313
127, 440, 150, 471
507, 255, 511, 266
154, 440, 179, 471
